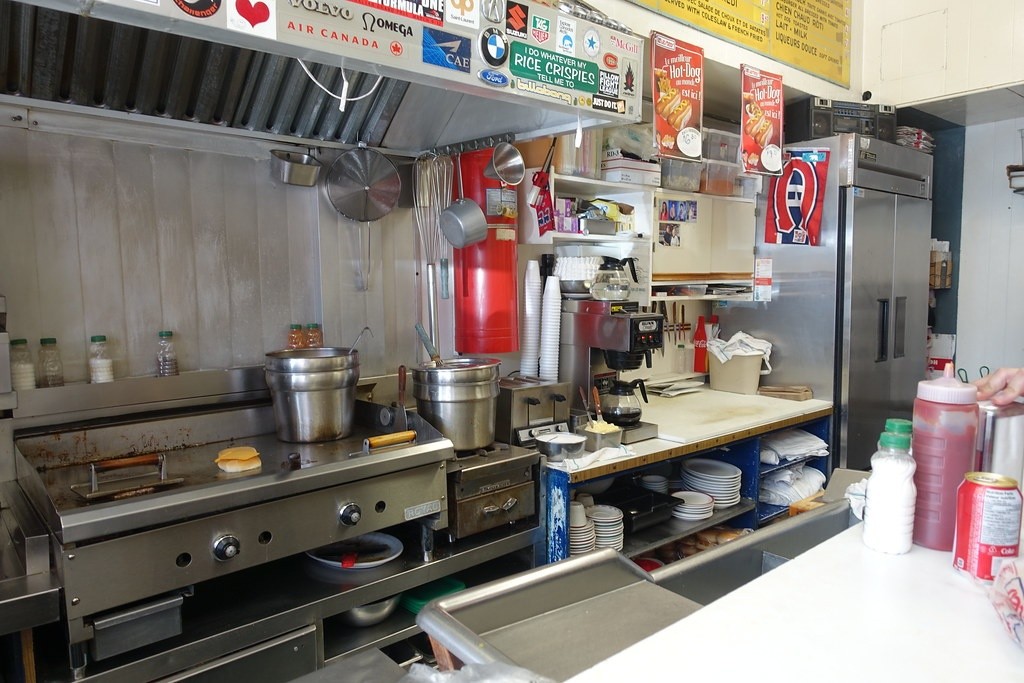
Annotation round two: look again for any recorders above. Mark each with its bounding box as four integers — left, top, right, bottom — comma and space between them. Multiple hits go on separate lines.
785, 98, 897, 143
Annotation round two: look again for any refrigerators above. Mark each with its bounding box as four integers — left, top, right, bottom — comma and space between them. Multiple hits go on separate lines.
709, 134, 934, 471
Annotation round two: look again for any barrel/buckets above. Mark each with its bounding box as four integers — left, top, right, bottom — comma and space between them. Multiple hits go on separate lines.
409, 357, 502, 450
263, 347, 359, 444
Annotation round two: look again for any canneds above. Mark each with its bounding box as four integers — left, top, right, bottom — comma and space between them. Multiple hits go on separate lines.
952, 472, 1023, 588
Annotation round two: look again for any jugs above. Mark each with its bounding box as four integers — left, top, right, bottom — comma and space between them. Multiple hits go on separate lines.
592, 255, 639, 300
602, 378, 648, 426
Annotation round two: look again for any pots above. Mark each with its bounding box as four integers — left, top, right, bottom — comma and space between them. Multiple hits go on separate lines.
439, 151, 487, 246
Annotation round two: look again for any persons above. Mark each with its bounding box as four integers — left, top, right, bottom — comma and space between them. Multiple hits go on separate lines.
686, 202, 692, 219
670, 204, 676, 220
972, 367, 1024, 405
661, 202, 668, 221
679, 204, 685, 220
663, 225, 678, 246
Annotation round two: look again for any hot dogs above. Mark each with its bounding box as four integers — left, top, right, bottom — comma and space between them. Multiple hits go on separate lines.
745, 110, 773, 149
655, 87, 693, 131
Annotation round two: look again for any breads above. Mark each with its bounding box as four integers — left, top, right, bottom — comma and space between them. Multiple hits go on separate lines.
638, 527, 751, 558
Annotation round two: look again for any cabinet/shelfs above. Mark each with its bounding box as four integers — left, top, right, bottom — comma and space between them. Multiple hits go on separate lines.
63, 414, 831, 683
517, 165, 759, 307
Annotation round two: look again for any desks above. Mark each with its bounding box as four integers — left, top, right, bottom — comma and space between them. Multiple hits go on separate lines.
562, 522, 1023, 683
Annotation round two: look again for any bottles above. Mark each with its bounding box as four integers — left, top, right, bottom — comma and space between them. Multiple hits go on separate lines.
305, 324, 322, 349
287, 324, 305, 348
860, 363, 979, 556
676, 316, 708, 375
709, 315, 719, 339
10, 330, 179, 391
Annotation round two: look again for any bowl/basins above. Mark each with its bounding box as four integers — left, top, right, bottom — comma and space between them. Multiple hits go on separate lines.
535, 432, 588, 462
270, 148, 324, 187
335, 592, 402, 627
560, 280, 589, 293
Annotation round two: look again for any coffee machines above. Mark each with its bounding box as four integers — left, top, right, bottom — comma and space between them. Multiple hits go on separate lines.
557, 299, 665, 445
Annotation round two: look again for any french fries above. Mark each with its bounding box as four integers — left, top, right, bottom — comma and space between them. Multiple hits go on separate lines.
658, 78, 670, 91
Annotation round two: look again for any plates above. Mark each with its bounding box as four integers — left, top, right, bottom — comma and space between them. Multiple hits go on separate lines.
304, 532, 404, 568
676, 128, 782, 172
561, 292, 593, 299
569, 459, 743, 559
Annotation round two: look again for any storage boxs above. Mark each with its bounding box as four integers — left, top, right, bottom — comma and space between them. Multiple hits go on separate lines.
601, 128, 758, 199
929, 250, 952, 289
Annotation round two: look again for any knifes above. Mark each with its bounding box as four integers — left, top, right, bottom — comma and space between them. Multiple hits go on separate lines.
652, 300, 685, 357
578, 385, 603, 428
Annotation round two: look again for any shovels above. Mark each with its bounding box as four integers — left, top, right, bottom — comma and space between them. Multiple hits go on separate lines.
393, 365, 408, 433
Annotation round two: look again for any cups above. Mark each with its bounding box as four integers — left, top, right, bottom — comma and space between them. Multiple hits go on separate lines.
569, 488, 593, 527
695, 322, 712, 372
520, 253, 602, 382
